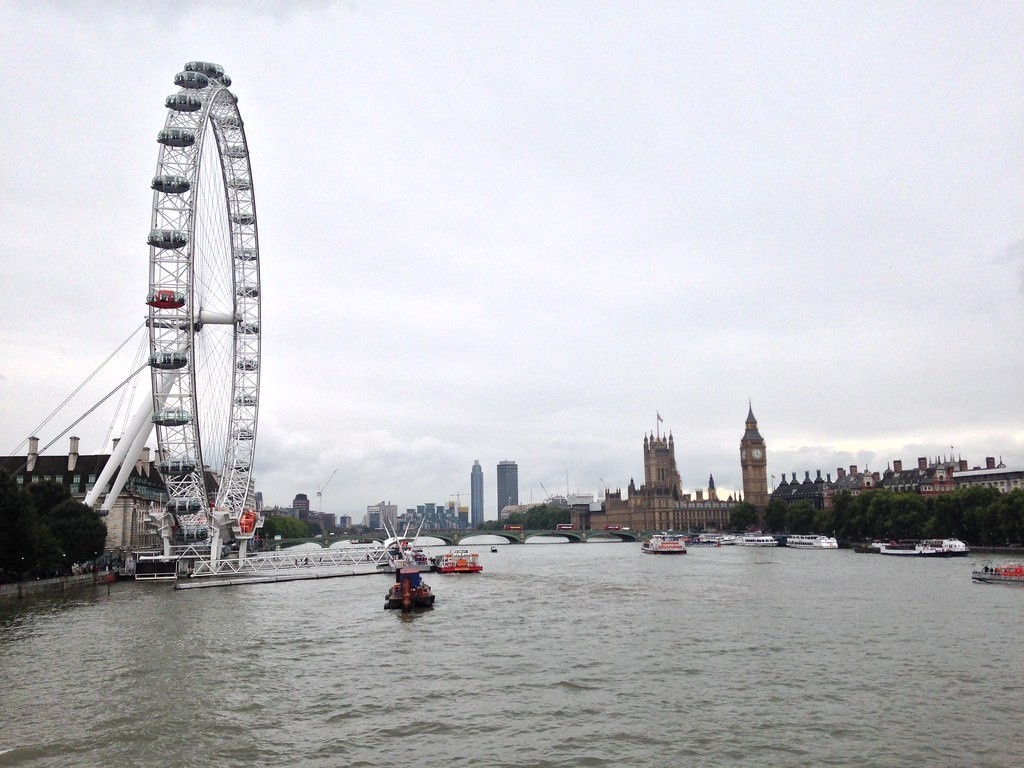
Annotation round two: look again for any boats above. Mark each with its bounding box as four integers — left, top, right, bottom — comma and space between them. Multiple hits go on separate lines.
737, 533, 779, 548
434, 548, 484, 573
641, 534, 688, 556
787, 534, 840, 550
491, 546, 497, 553
878, 536, 971, 557
388, 567, 435, 613
971, 562, 1024, 584
698, 531, 740, 546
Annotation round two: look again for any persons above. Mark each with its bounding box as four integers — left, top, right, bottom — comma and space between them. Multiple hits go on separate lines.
983, 565, 993, 575
430, 557, 436, 564
295, 557, 307, 566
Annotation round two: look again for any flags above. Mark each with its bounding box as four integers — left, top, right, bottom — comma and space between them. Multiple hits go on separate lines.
658, 413, 663, 422
